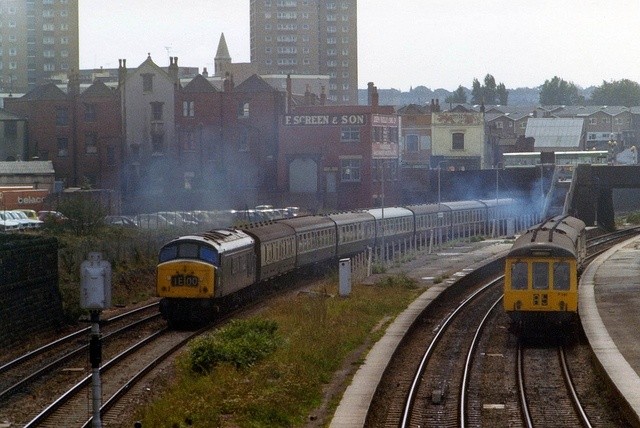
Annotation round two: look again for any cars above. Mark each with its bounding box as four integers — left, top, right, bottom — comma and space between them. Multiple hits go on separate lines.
130, 213, 176, 230
0, 208, 38, 231
175, 204, 300, 228
157, 213, 175, 222
104, 215, 137, 232
38, 210, 70, 224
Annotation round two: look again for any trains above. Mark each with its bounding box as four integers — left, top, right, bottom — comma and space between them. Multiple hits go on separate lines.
154, 197, 520, 323
503, 214, 586, 329
